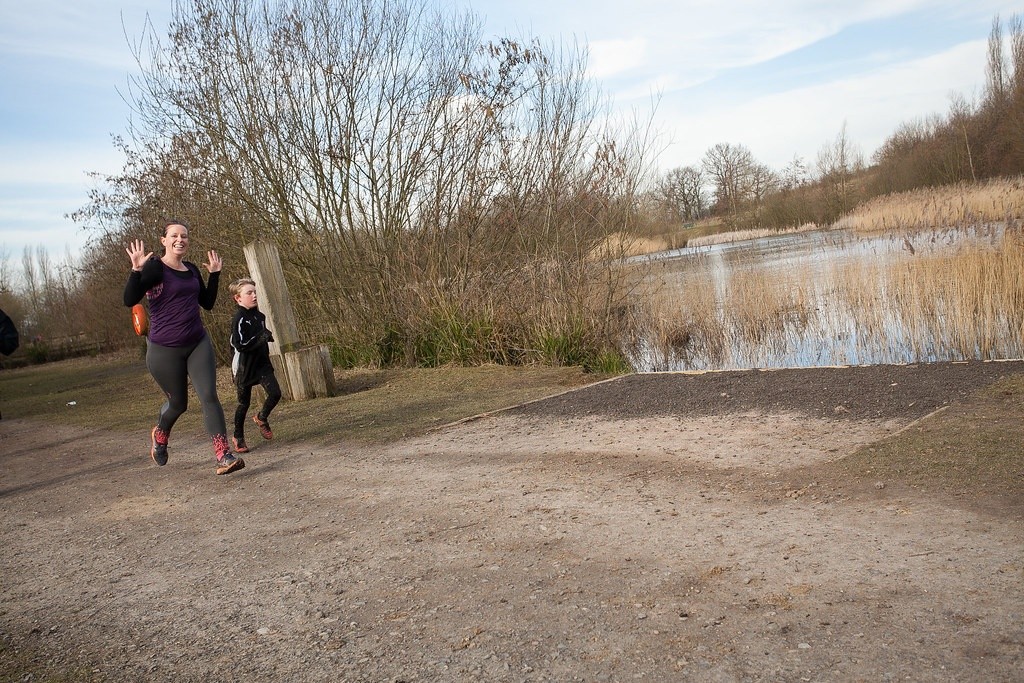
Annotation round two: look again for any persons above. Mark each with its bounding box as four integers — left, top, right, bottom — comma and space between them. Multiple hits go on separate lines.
228, 279, 283, 453
0, 309, 20, 355
123, 221, 246, 476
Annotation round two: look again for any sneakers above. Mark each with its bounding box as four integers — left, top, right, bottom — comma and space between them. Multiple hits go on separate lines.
252, 412, 273, 440
232, 436, 249, 453
216, 449, 245, 475
152, 426, 168, 467
